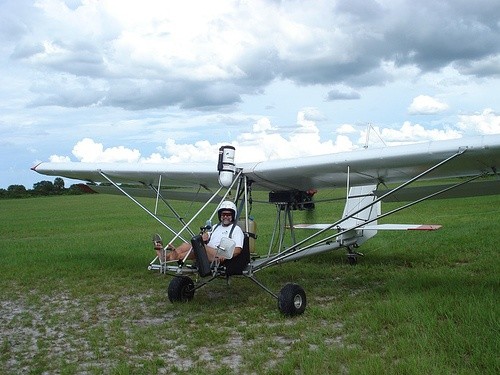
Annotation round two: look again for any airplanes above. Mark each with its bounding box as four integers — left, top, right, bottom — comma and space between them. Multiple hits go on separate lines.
27, 132, 500, 316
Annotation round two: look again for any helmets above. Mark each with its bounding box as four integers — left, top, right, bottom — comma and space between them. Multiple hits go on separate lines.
218, 200, 237, 222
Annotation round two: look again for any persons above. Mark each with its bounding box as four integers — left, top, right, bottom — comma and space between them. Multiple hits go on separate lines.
153, 201, 244, 266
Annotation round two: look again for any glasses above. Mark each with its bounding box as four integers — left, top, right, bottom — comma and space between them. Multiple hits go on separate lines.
220, 213, 233, 216
